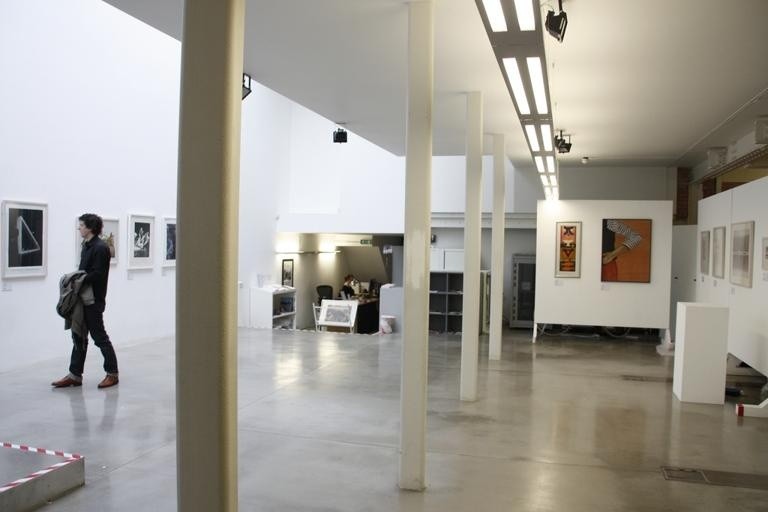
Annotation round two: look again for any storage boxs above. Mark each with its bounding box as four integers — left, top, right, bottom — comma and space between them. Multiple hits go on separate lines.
429, 247, 444, 271
443, 248, 463, 273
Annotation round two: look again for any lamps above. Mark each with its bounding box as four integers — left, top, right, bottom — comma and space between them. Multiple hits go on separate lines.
544, 2, 570, 46
552, 128, 573, 157
330, 121, 350, 144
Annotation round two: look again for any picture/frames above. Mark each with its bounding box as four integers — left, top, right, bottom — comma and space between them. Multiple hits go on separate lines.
729, 222, 754, 288
281, 259, 293, 288
162, 217, 176, 267
699, 229, 709, 276
554, 220, 580, 279
0, 200, 49, 278
74, 215, 120, 266
601, 218, 650, 284
710, 226, 726, 279
126, 212, 156, 271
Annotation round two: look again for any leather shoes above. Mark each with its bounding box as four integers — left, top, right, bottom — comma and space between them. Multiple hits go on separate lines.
51, 377, 82, 386
98, 375, 120, 388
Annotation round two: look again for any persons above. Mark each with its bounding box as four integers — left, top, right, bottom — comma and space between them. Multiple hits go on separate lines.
338, 275, 359, 300
50, 214, 119, 387
602, 219, 642, 281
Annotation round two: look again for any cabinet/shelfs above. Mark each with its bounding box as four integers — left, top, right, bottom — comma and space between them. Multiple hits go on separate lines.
429, 272, 447, 331
444, 273, 461, 332
250, 285, 296, 329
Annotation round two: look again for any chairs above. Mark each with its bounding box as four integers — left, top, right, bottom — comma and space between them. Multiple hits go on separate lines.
315, 285, 333, 306
